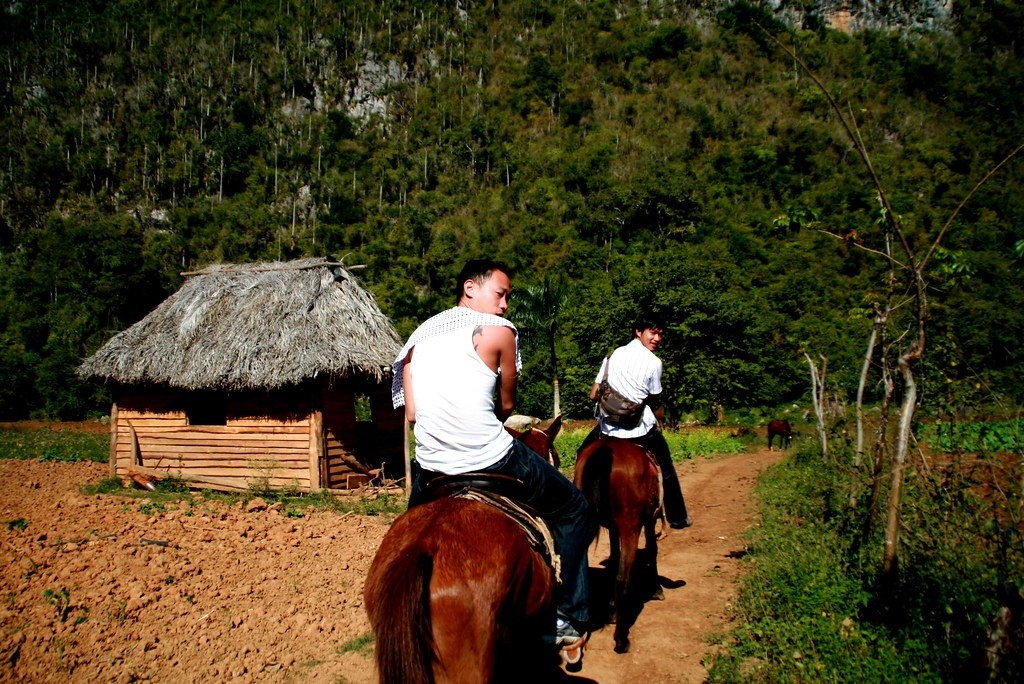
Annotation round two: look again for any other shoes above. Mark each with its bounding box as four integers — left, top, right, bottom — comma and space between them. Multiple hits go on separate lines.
670, 515, 693, 530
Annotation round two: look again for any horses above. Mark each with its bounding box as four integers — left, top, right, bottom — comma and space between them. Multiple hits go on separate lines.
362, 413, 568, 684
572, 401, 666, 651
767, 420, 793, 451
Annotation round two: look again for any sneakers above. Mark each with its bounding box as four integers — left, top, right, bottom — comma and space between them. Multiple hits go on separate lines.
543, 615, 580, 645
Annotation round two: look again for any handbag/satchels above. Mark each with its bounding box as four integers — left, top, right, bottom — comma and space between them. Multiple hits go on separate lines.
595, 382, 642, 431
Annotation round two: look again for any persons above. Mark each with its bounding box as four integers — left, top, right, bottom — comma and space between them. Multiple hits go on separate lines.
403, 260, 599, 641
577, 312, 693, 529
